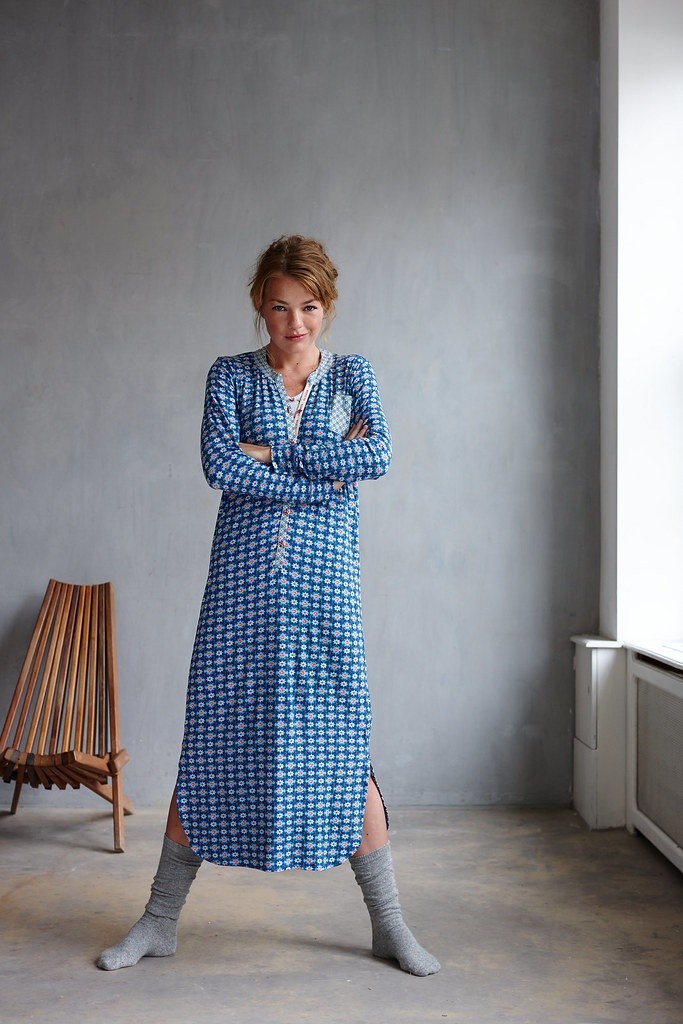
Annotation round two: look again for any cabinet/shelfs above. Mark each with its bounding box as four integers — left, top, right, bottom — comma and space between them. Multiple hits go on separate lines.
568, 633, 626, 830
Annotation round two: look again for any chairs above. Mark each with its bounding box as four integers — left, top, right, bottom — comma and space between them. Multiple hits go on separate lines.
0, 578, 135, 853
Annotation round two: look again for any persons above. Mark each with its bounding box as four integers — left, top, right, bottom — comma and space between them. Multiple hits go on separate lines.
95, 235, 440, 977
